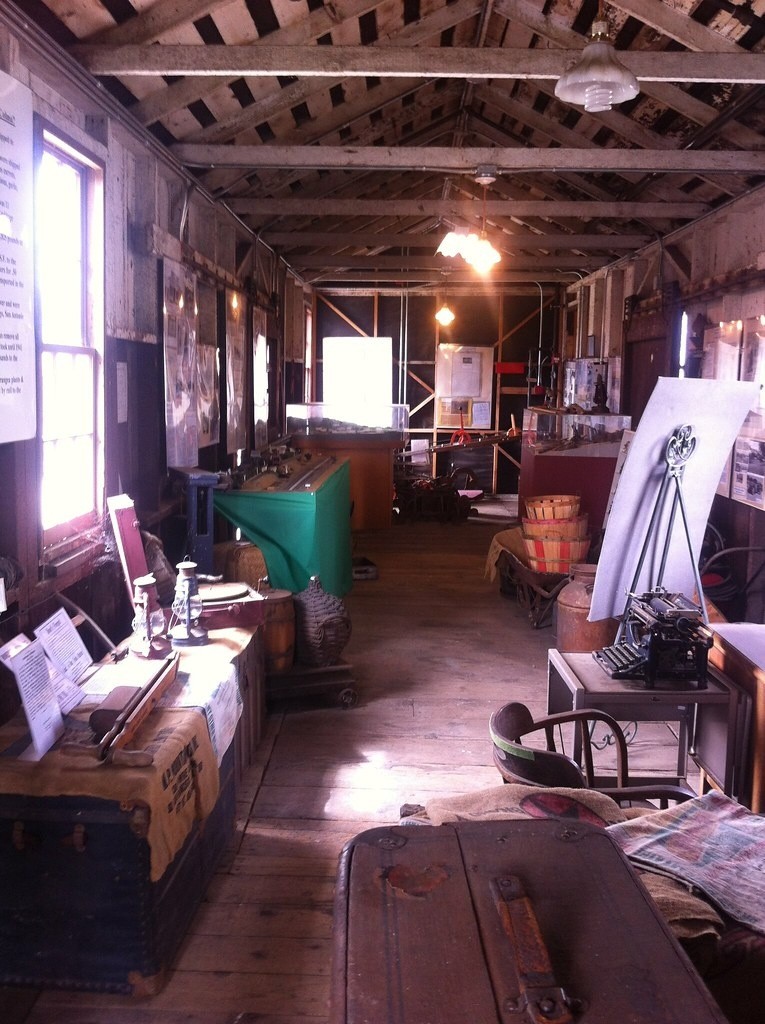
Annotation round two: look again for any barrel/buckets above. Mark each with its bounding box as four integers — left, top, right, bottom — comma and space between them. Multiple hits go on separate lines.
255, 588, 295, 675
522, 495, 591, 576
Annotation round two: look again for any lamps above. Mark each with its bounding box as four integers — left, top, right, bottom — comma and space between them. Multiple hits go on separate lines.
555, 8, 640, 114
170, 555, 209, 645
435, 172, 502, 325
131, 573, 173, 660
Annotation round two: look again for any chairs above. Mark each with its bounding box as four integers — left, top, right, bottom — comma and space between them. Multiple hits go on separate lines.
692, 522, 765, 625
489, 703, 701, 810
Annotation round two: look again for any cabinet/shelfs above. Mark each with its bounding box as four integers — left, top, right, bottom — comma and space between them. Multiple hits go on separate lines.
514, 410, 632, 527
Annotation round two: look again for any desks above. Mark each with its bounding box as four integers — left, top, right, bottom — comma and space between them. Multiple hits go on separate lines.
704, 620, 765, 817
545, 650, 753, 801
214, 456, 355, 599
0, 631, 268, 956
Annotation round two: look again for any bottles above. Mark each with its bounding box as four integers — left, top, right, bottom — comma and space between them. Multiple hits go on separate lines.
215, 444, 313, 490
